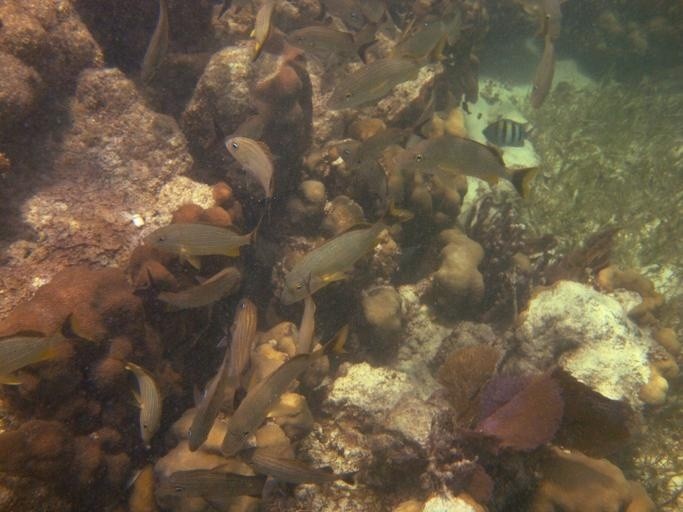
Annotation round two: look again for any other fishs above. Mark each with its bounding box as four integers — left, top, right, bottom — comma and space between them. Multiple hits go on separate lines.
1, 0, 566, 512
